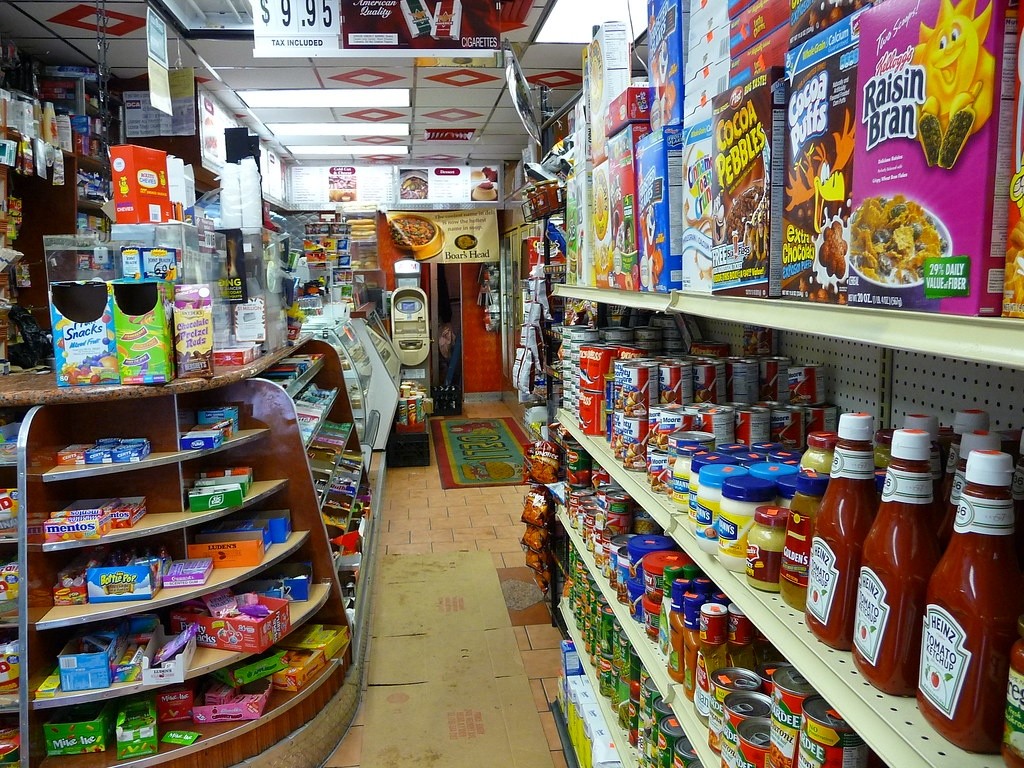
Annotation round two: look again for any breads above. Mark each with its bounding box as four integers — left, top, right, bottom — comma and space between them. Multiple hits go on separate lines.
347, 219, 376, 239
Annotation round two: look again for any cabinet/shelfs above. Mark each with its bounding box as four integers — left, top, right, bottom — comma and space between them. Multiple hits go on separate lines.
0, 98, 110, 360
553, 282, 1024, 768
302, 315, 381, 475
0, 331, 371, 768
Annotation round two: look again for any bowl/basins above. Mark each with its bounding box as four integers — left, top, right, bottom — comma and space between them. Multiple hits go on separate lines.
848, 199, 952, 287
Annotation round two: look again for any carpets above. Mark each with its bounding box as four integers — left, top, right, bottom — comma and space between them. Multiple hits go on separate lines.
430, 417, 532, 490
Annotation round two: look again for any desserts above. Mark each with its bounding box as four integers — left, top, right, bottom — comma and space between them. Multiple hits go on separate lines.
471, 170, 486, 181
472, 182, 497, 200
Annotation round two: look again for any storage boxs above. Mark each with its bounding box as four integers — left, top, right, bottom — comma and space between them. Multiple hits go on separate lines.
122, 245, 179, 282
564, 0, 1024, 318
213, 348, 254, 366
109, 276, 176, 386
109, 143, 174, 224
387, 414, 431, 468
227, 343, 263, 360
44, 406, 352, 760
47, 278, 121, 387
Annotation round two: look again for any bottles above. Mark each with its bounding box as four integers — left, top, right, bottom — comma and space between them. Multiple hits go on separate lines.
777, 409, 1024, 768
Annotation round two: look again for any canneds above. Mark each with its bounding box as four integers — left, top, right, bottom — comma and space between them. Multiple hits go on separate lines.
564, 325, 873, 767
